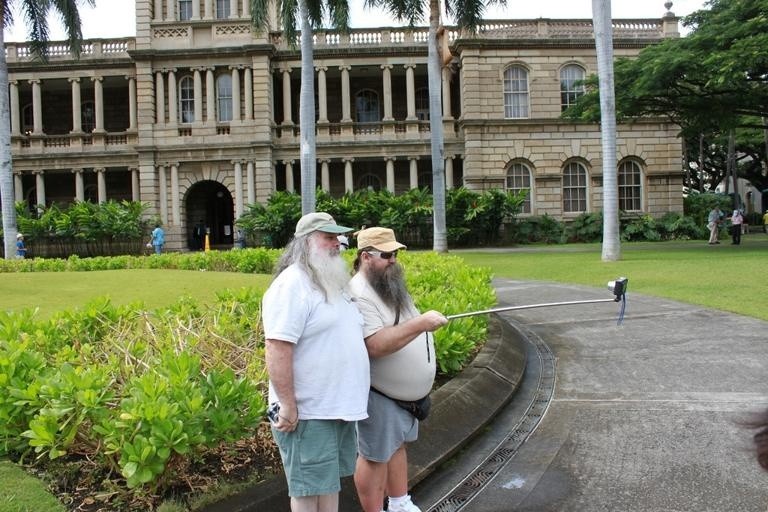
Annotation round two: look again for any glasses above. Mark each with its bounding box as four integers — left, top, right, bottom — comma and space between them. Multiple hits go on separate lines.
368, 251, 398, 258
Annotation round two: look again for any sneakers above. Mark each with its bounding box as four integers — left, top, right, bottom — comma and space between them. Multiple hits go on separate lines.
709, 241, 739, 244
388, 494, 421, 511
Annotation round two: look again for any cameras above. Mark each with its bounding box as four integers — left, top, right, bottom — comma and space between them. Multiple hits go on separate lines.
266, 402, 281, 423
607, 277, 628, 295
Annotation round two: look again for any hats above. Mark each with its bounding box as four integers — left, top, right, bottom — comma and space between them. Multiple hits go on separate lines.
294, 213, 353, 237
17, 234, 24, 238
357, 226, 406, 254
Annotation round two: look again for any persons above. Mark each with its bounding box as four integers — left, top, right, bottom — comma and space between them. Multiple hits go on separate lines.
707, 202, 720, 245
707, 204, 724, 239
725, 209, 743, 246
762, 209, 768, 233
147, 222, 164, 255
259, 210, 382, 512
343, 220, 450, 512
191, 218, 204, 251
15, 232, 28, 258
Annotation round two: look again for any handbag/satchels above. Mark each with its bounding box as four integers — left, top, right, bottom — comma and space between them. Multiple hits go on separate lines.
397, 396, 431, 419
